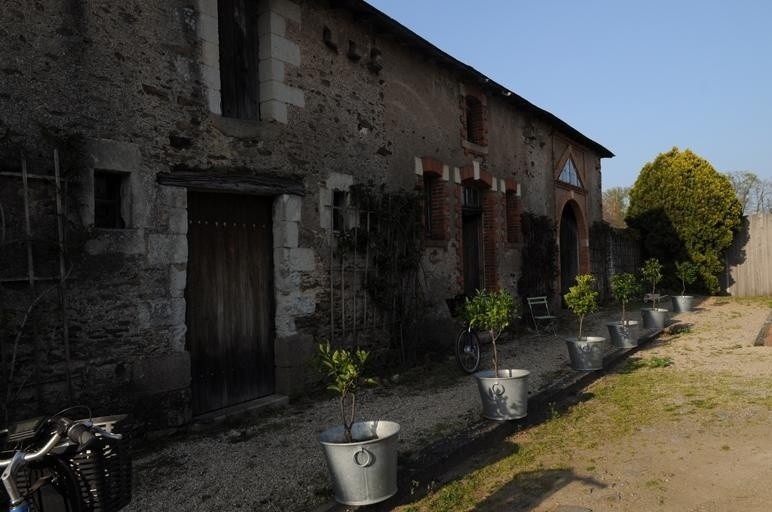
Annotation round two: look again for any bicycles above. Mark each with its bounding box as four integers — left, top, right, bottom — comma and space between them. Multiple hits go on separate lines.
454, 316, 483, 375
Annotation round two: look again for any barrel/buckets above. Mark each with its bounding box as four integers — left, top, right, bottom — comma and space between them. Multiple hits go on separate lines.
318, 419, 399, 506
672, 295, 694, 312
642, 308, 669, 328
474, 367, 530, 421
565, 336, 606, 370
608, 320, 640, 347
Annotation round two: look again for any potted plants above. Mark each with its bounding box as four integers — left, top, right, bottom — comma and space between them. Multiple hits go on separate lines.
607, 269, 640, 349
640, 257, 668, 329
564, 274, 605, 371
671, 260, 699, 314
315, 339, 402, 507
455, 289, 531, 420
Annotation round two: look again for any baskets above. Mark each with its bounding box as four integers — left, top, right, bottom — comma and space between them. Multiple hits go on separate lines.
445, 298, 468, 317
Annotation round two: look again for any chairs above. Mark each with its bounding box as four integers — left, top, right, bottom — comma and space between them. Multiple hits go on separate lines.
527, 296, 557, 343
